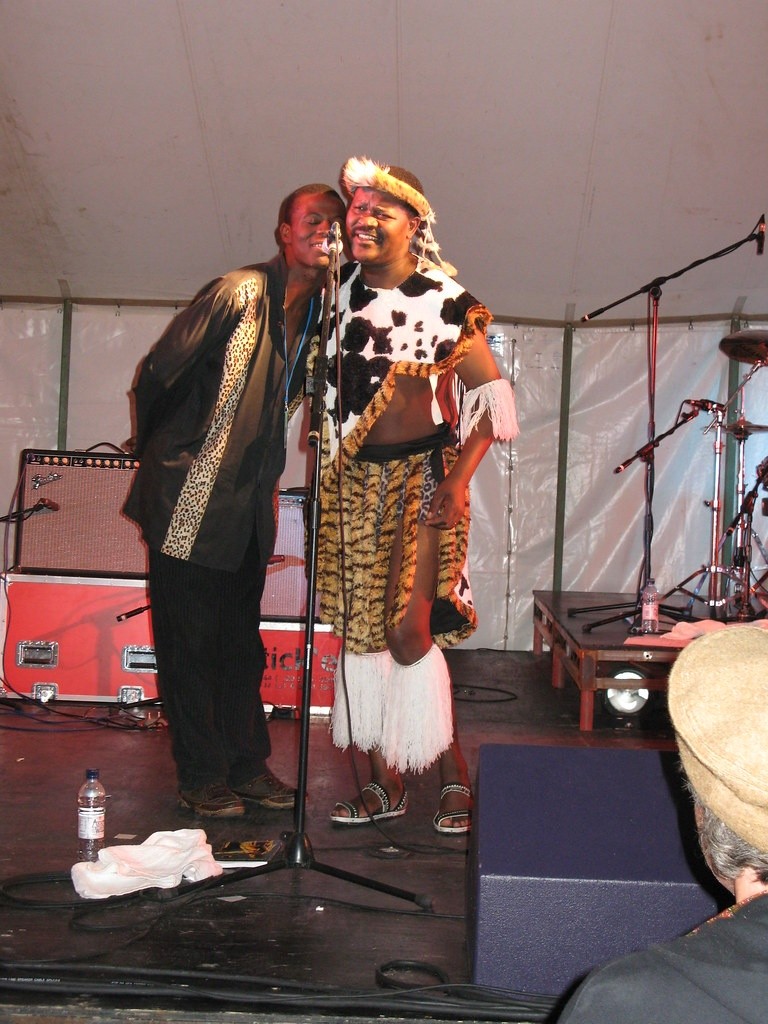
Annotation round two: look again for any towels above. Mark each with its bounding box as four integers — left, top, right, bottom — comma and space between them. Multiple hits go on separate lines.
70, 827, 225, 900
659, 619, 768, 642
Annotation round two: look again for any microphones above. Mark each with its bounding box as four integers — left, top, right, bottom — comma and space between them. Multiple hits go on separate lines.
41, 497, 60, 511
757, 216, 767, 255
685, 399, 727, 411
267, 555, 284, 564
322, 222, 344, 255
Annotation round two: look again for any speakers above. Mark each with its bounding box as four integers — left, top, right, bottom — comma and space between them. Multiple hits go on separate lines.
259, 488, 323, 624
17, 449, 154, 578
465, 744, 737, 996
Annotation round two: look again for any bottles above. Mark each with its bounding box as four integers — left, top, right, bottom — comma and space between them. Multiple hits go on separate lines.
640, 578, 659, 634
77, 768, 106, 862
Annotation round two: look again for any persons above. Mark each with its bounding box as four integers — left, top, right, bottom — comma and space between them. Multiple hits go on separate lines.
308, 158, 518, 834
557, 624, 767, 1024
123, 185, 345, 818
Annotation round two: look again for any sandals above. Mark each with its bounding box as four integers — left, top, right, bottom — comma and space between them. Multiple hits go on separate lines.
331, 781, 409, 823
434, 784, 472, 833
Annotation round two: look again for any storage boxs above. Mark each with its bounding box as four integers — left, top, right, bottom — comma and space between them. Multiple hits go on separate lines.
0, 573, 339, 717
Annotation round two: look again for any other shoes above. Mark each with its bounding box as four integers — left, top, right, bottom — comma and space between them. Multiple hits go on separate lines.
233, 773, 309, 809
179, 783, 246, 818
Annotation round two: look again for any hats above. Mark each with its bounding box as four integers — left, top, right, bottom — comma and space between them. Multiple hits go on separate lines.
668, 626, 768, 853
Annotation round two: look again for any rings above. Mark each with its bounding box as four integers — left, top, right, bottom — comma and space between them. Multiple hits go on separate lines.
454, 522, 456, 524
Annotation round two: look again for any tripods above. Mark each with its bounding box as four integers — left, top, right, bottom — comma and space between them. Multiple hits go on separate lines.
158, 250, 439, 913
566, 233, 768, 635
